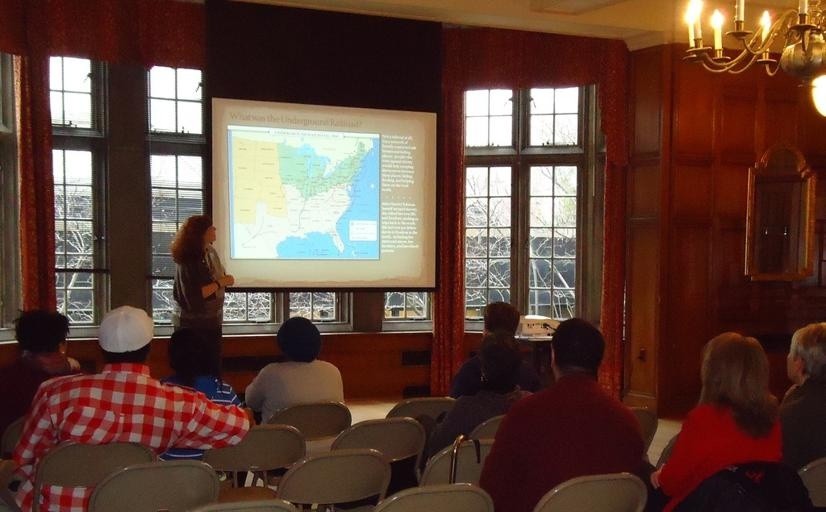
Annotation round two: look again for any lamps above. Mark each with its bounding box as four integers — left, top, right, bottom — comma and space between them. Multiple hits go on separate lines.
673, 0, 825, 93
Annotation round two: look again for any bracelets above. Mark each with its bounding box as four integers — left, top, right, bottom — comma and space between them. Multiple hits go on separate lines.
215, 279, 220, 289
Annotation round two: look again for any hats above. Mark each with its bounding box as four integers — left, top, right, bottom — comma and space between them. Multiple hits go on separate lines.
99, 305, 154, 353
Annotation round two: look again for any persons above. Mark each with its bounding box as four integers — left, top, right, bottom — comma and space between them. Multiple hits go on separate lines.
159, 327, 245, 463
12, 306, 253, 512
244, 315, 346, 476
644, 333, 785, 511
170, 214, 235, 386
415, 330, 535, 474
479, 316, 647, 511
448, 301, 548, 399
777, 323, 826, 470
0, 308, 85, 429
